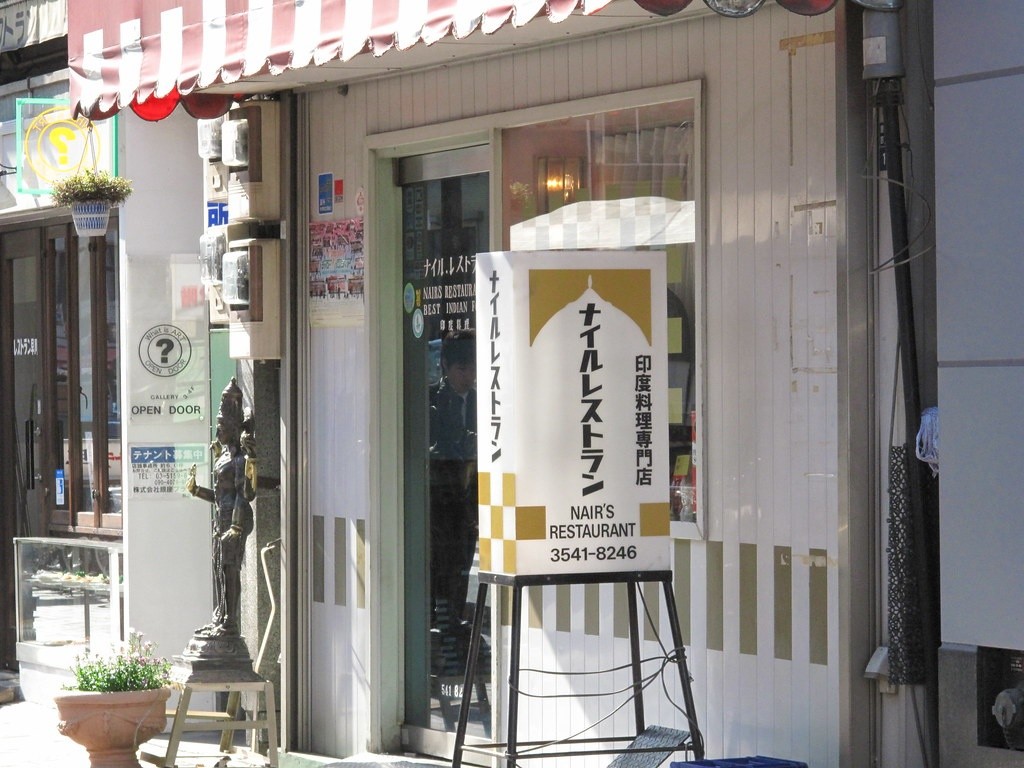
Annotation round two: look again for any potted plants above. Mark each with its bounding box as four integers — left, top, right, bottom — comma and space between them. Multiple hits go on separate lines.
51, 169, 135, 240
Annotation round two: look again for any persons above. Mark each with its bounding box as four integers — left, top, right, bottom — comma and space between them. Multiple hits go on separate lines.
428, 329, 482, 638
188, 374, 255, 639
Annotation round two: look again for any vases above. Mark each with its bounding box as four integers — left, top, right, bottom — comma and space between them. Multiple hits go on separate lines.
54, 687, 172, 767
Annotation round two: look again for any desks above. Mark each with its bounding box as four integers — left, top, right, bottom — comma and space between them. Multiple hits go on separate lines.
453, 569, 703, 768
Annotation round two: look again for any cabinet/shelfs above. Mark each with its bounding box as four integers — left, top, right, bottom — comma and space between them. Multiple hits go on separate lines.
14, 538, 126, 649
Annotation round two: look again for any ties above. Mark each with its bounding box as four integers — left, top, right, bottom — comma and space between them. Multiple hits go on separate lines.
455, 395, 464, 445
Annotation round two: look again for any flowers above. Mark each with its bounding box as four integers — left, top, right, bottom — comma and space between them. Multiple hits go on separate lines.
66, 626, 176, 693
